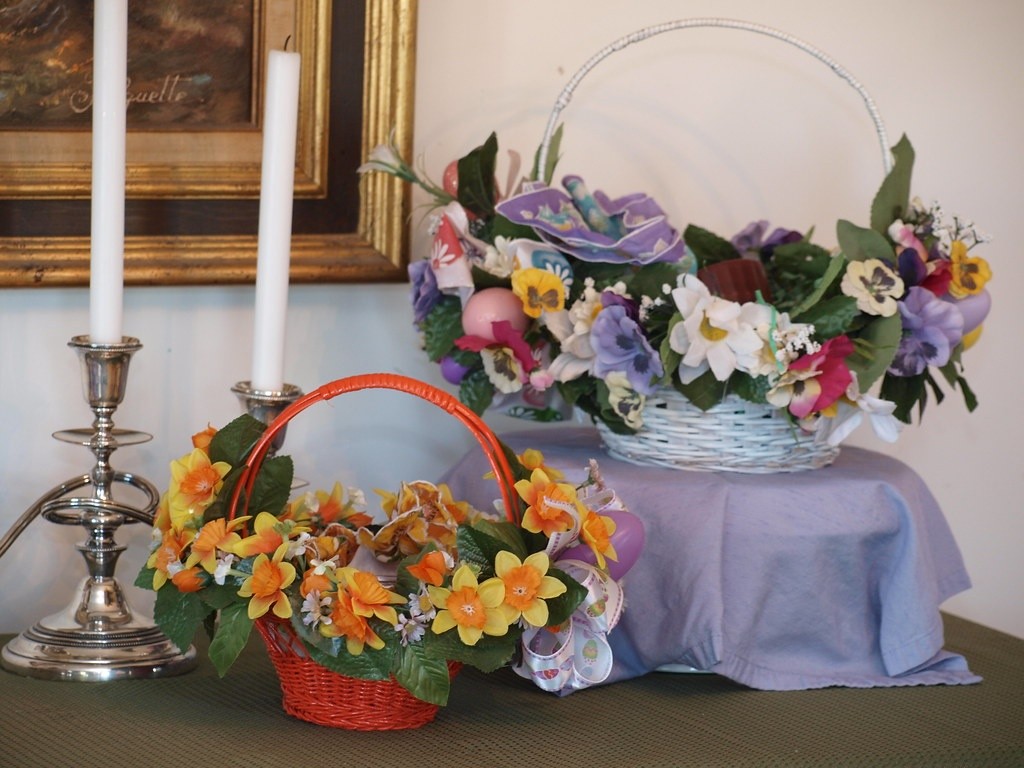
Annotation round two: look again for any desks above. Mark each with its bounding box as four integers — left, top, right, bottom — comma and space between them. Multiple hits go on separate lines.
0, 603, 1024, 768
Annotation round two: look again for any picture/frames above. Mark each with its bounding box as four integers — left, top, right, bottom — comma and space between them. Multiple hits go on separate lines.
0, 0, 417, 284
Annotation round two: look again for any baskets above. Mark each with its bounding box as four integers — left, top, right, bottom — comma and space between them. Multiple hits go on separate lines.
537, 21, 892, 474
227, 374, 521, 729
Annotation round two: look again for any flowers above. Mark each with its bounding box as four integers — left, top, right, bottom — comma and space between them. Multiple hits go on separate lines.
361, 128, 994, 451
132, 413, 624, 715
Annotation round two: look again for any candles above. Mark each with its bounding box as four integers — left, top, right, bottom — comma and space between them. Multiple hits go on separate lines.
87, 0, 129, 345
252, 34, 302, 392
